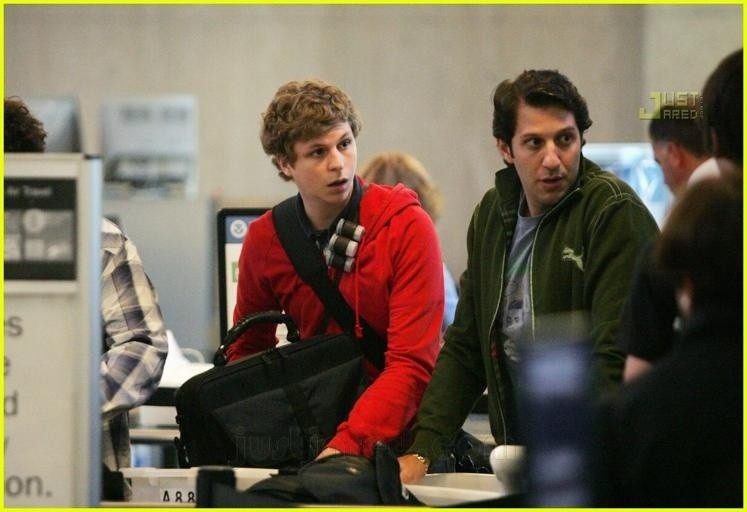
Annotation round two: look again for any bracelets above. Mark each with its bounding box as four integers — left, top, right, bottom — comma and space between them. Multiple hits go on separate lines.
412, 454, 429, 474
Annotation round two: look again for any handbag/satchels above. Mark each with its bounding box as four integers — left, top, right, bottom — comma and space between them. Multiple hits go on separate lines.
174, 327, 370, 472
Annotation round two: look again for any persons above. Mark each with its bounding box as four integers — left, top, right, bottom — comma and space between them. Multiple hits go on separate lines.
360, 153, 457, 332
400, 70, 659, 508
622, 49, 743, 385
4, 93, 169, 501
601, 173, 743, 509
223, 79, 445, 463
650, 104, 711, 195
